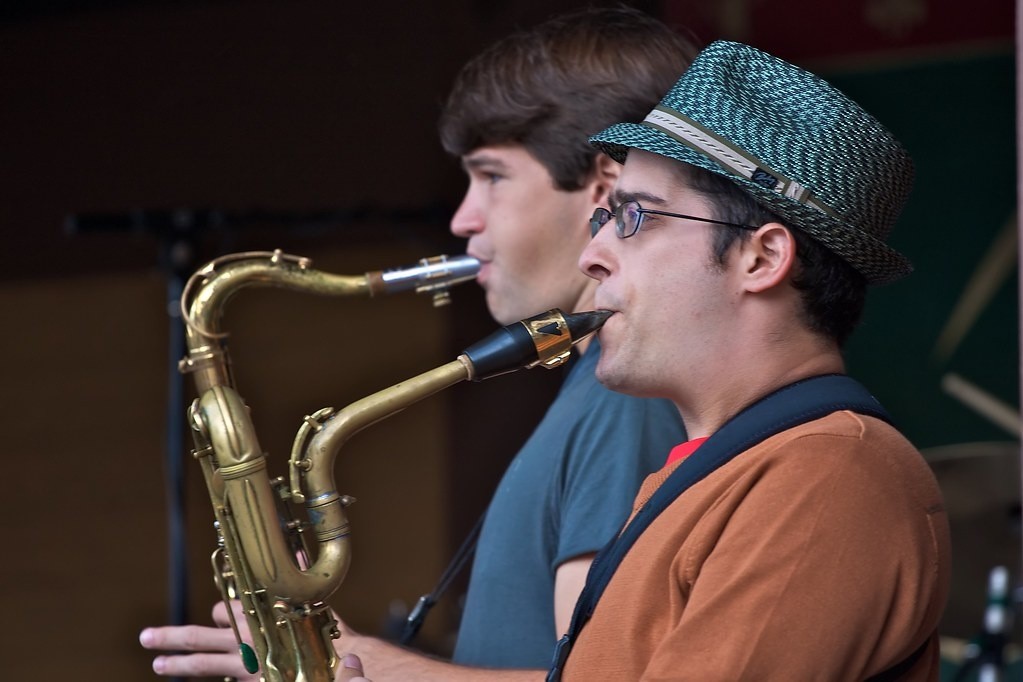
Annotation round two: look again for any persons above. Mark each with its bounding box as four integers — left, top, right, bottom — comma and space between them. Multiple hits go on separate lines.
140, 13, 698, 682
329, 39, 950, 682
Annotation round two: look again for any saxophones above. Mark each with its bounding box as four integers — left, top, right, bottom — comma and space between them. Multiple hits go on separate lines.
190, 306, 617, 682
178, 249, 483, 600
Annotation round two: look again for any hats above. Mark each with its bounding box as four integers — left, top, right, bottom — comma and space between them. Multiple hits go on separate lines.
590, 40, 913, 284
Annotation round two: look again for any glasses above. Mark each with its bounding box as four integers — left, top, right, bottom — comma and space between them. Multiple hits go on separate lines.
590, 201, 759, 238
